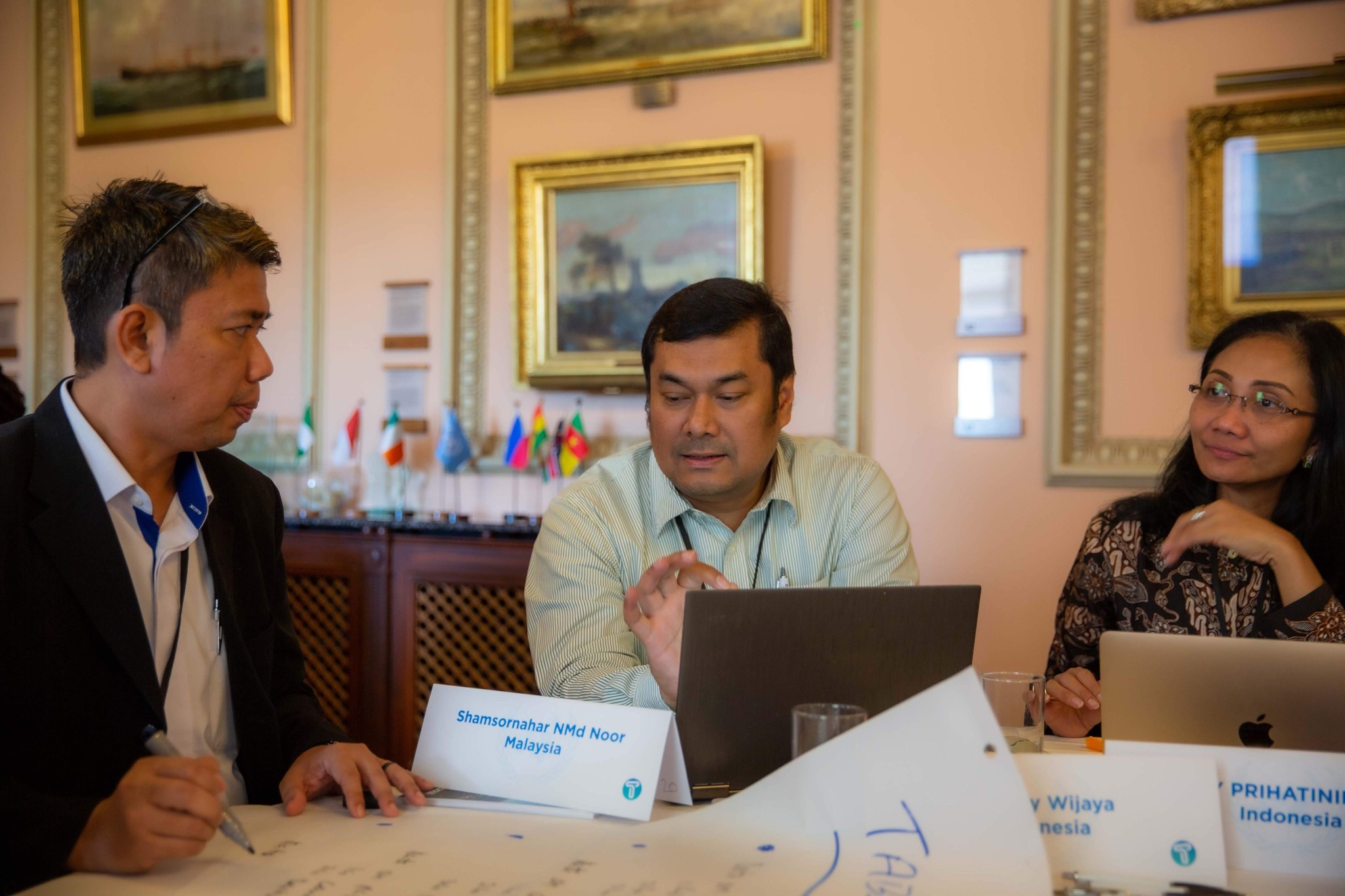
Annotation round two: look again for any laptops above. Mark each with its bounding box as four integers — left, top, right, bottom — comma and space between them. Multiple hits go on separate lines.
1101, 630, 1345, 755
676, 584, 981, 799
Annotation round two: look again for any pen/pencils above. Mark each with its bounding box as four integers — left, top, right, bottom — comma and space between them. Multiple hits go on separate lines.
214, 599, 223, 655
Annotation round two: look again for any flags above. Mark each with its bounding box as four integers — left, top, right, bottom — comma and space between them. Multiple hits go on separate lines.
502, 399, 592, 483
379, 406, 404, 468
292, 402, 315, 464
346, 409, 359, 452
434, 406, 471, 475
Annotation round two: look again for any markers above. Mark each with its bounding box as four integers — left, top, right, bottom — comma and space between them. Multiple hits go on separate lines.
1086, 736, 1103, 753
140, 723, 254, 858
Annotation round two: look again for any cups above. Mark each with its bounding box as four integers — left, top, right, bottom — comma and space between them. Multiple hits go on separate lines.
791, 703, 868, 759
982, 670, 1044, 755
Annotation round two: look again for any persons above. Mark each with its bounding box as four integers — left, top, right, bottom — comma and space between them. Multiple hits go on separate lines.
1025, 308, 1345, 737
1, 179, 437, 896
523, 279, 920, 712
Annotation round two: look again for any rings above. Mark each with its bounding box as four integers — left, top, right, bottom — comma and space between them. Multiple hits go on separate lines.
1191, 505, 1205, 520
381, 761, 394, 772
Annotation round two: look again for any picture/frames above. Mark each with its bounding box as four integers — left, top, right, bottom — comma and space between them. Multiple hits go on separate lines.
511, 135, 766, 388
68, 0, 294, 145
485, 0, 832, 96
1188, 89, 1345, 351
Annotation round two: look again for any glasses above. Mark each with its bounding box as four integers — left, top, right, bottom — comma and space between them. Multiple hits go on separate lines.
1189, 380, 1317, 420
122, 189, 225, 309
